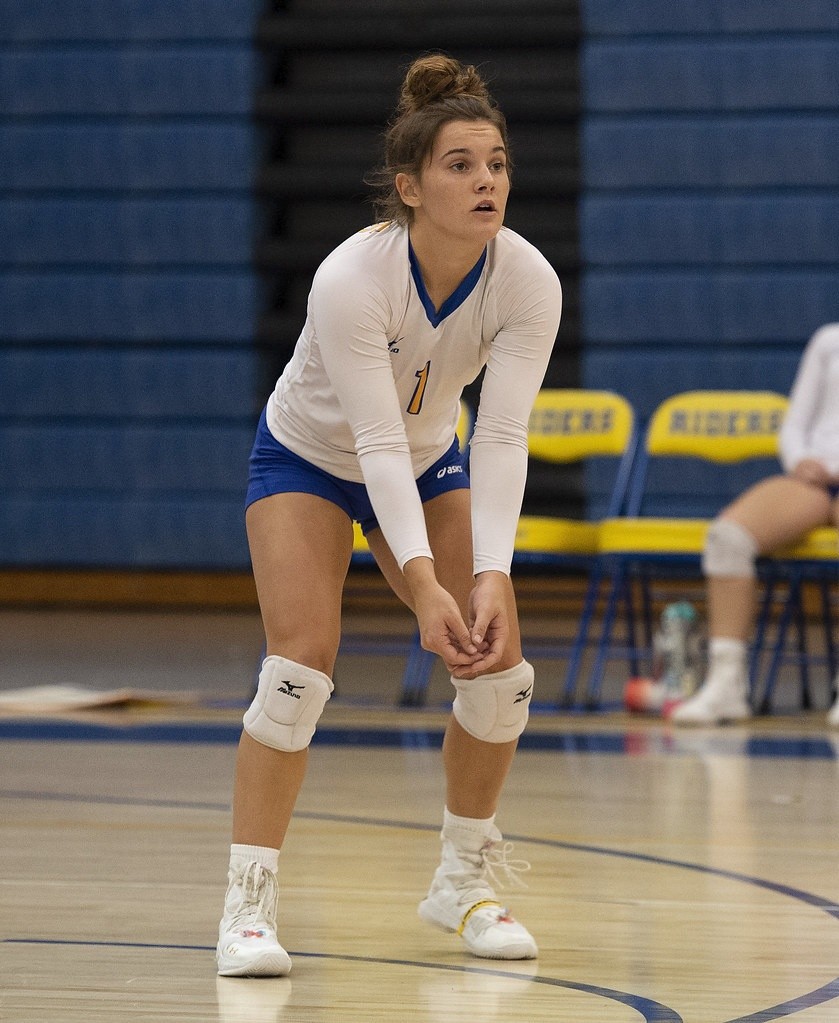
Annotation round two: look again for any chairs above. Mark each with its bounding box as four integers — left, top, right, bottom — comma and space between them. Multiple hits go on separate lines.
250, 386, 839, 718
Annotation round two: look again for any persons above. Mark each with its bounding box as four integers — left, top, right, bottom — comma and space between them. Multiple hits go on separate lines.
214, 57, 563, 977
669, 322, 839, 727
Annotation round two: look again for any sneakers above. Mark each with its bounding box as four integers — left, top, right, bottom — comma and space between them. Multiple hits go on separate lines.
419, 825, 540, 959
673, 648, 750, 724
214, 860, 291, 978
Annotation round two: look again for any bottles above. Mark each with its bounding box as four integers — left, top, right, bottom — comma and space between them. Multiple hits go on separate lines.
625, 599, 703, 714
